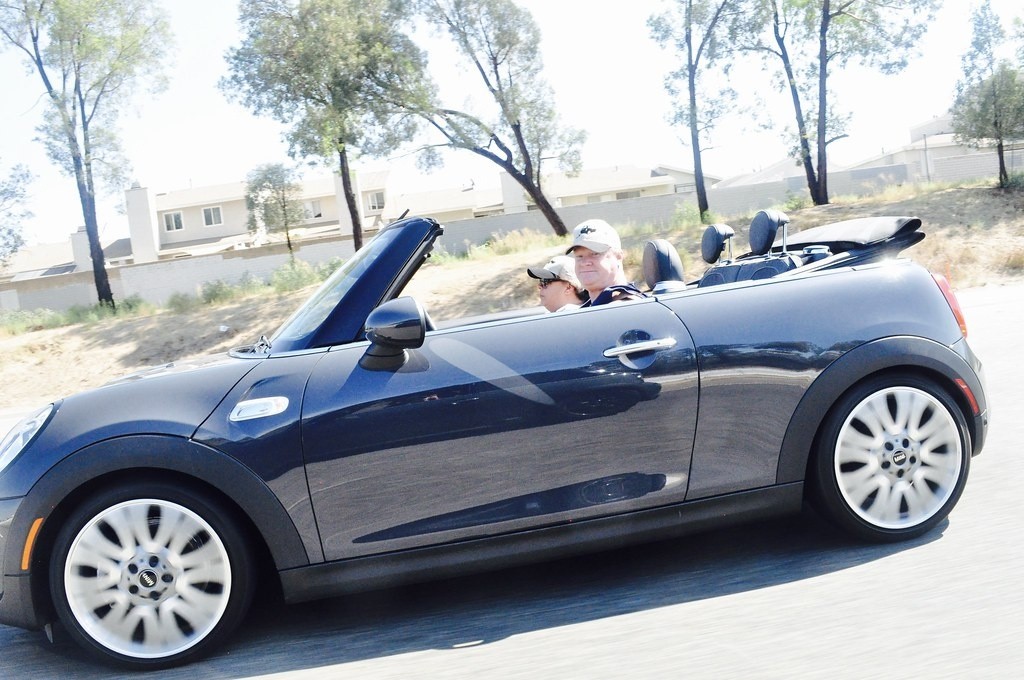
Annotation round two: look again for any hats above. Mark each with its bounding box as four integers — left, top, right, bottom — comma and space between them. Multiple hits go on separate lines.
528, 255, 581, 289
565, 220, 621, 254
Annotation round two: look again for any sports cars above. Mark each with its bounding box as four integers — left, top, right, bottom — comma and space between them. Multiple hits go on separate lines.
1, 206, 989, 671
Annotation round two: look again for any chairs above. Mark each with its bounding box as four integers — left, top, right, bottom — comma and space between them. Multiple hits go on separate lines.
640, 238, 690, 293
701, 211, 802, 285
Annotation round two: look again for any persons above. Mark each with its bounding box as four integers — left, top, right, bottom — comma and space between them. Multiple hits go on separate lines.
565, 219, 649, 308
527, 255, 591, 313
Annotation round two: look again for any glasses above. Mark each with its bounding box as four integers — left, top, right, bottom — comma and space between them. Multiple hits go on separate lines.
539, 278, 563, 287
